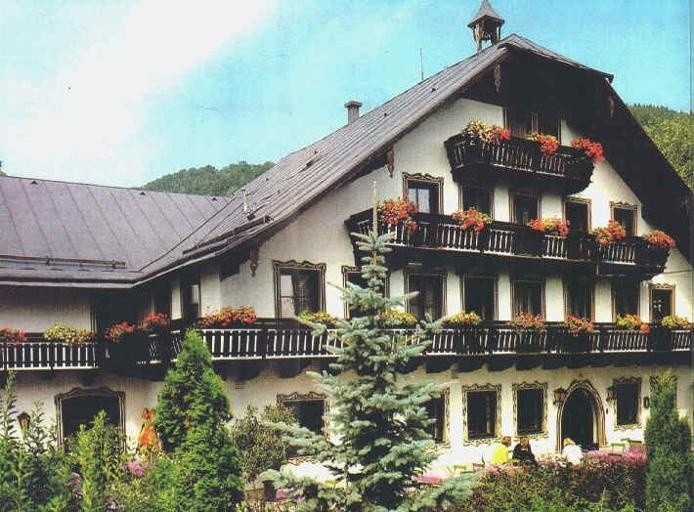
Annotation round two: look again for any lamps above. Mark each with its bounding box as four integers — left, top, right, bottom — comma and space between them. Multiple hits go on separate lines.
605, 382, 620, 403
552, 384, 569, 407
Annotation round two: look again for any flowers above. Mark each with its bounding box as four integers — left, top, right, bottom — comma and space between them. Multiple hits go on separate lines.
661, 314, 692, 330
296, 309, 340, 326
643, 230, 677, 249
462, 120, 512, 141
381, 309, 416, 325
107, 323, 135, 338
444, 311, 483, 326
530, 130, 559, 153
452, 207, 493, 232
140, 312, 170, 329
616, 314, 650, 334
44, 324, 95, 345
508, 311, 550, 331
592, 219, 627, 247
1, 326, 29, 346
376, 196, 419, 229
201, 305, 257, 325
563, 314, 596, 334
572, 136, 603, 163
526, 216, 569, 237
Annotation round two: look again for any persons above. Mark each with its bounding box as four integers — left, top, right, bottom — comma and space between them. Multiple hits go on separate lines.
512, 435, 535, 463
560, 436, 583, 466
490, 435, 512, 466
